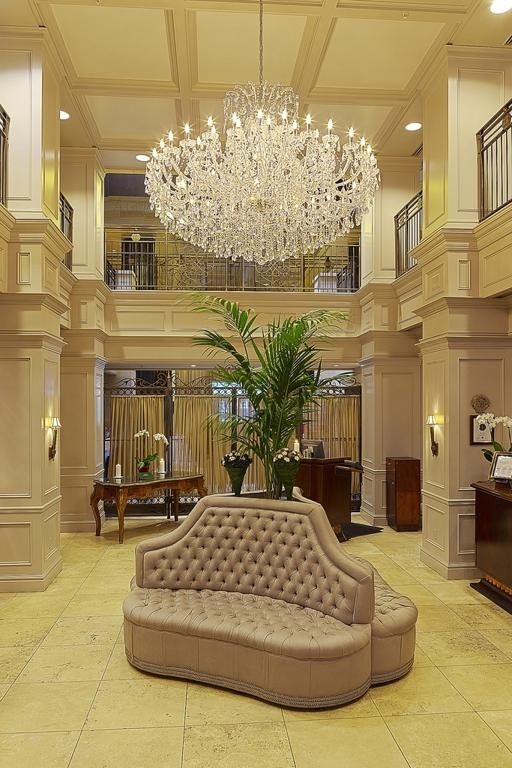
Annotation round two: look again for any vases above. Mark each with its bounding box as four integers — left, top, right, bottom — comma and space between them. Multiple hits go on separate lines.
137, 462, 149, 472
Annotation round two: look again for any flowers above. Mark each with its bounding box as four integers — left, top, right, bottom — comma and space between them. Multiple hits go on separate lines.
134, 429, 169, 466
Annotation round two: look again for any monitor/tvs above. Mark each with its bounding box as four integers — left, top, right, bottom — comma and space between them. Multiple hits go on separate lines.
301, 439, 325, 458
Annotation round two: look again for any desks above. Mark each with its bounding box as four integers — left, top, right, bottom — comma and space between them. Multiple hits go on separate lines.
469, 481, 512, 614
90, 471, 208, 544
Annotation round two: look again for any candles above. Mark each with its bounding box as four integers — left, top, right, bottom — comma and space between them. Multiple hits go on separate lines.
159, 458, 165, 478
116, 464, 122, 484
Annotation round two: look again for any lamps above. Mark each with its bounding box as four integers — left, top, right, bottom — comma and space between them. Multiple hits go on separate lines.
144, 0, 381, 266
425, 415, 438, 456
49, 417, 63, 460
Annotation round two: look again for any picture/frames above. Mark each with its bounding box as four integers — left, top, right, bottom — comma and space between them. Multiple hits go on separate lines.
488, 450, 512, 480
469, 414, 496, 445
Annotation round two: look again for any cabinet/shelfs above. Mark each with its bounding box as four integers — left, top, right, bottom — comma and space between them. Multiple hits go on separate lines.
386, 456, 421, 532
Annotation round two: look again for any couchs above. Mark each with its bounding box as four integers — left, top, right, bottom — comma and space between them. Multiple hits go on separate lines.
123, 486, 419, 709
